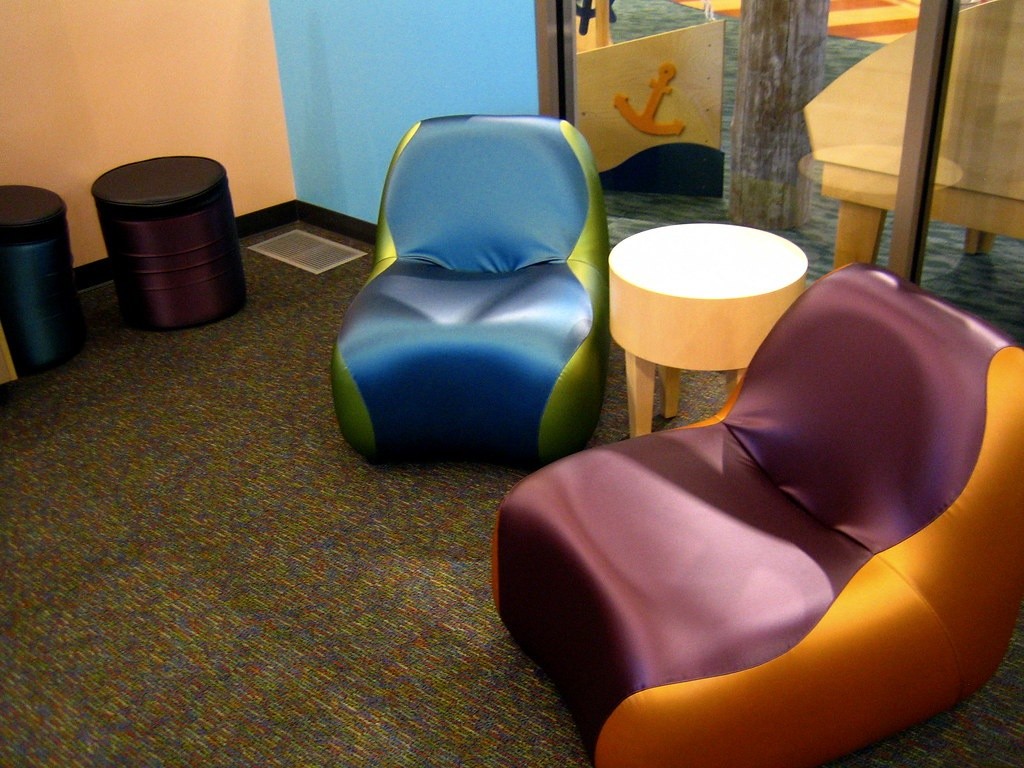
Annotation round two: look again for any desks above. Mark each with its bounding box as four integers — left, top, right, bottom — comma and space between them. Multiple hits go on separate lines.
608, 224, 808, 438
797, 0, 1024, 272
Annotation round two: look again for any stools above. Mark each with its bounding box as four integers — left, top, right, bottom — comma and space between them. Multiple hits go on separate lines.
0, 185, 91, 384
92, 156, 248, 329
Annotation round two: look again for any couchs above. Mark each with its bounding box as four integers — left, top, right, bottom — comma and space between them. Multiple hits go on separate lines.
492, 253, 1024, 767
333, 114, 609, 468
